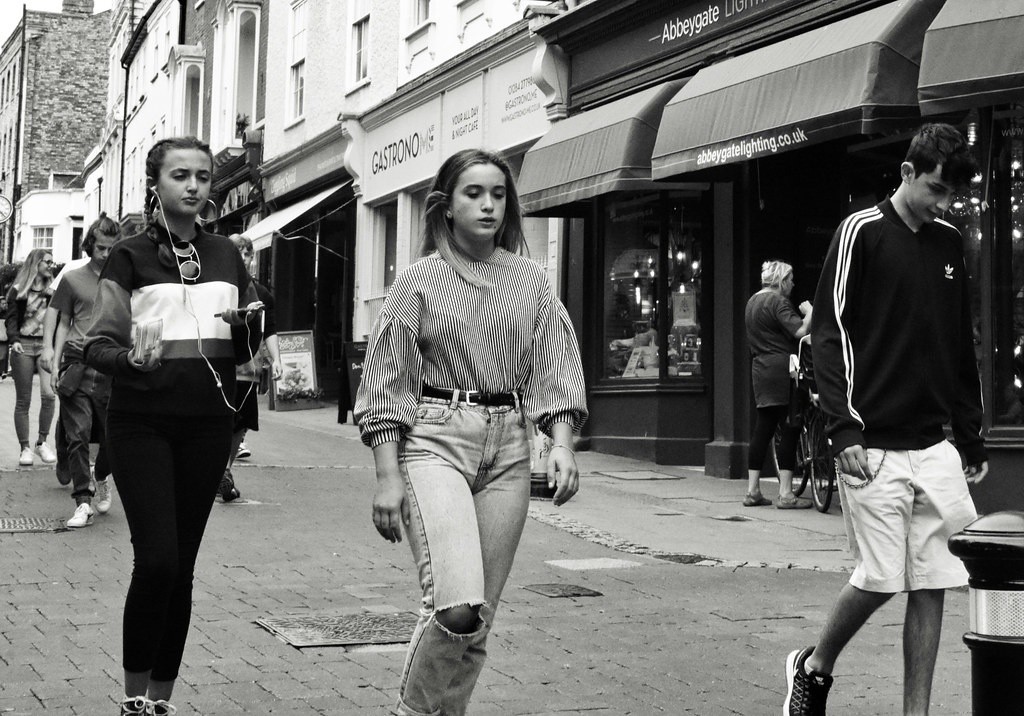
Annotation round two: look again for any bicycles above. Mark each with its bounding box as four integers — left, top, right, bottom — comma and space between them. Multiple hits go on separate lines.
769, 405, 836, 513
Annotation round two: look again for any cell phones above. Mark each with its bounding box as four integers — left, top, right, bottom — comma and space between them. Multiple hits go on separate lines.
214, 304, 267, 318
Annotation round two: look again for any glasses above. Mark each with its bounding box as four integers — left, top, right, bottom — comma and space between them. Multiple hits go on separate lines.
42, 259, 53, 266
173, 239, 201, 282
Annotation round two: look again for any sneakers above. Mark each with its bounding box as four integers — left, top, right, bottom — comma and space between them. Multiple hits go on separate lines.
782, 646, 833, 716
34, 441, 56, 462
67, 504, 91, 527
19, 447, 33, 465
91, 465, 111, 513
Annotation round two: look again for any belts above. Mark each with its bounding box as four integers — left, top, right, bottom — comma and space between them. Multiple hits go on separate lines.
423, 384, 522, 407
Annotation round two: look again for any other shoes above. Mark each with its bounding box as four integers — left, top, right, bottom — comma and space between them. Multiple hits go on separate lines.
777, 494, 813, 509
235, 446, 250, 458
218, 475, 239, 501
743, 493, 772, 507
120, 697, 175, 716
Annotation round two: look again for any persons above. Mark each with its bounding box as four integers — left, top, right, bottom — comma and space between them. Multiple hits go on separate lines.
781, 124, 989, 716
40, 210, 282, 528
81, 137, 265, 716
354, 149, 589, 716
742, 262, 813, 509
7, 248, 57, 465
0, 296, 7, 380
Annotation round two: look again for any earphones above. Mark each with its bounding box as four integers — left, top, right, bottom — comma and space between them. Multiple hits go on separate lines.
151, 185, 158, 195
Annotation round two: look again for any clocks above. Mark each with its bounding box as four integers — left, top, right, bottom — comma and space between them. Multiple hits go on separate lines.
0, 195, 13, 223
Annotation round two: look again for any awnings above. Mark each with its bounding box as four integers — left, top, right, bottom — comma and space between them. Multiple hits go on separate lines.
651, 0, 936, 183
514, 74, 710, 218
242, 174, 353, 252
917, 0, 1024, 116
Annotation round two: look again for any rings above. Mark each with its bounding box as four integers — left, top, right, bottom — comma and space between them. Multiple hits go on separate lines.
551, 444, 577, 456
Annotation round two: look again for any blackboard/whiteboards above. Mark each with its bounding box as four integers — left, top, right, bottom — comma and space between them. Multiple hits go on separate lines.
338, 341, 368, 411
